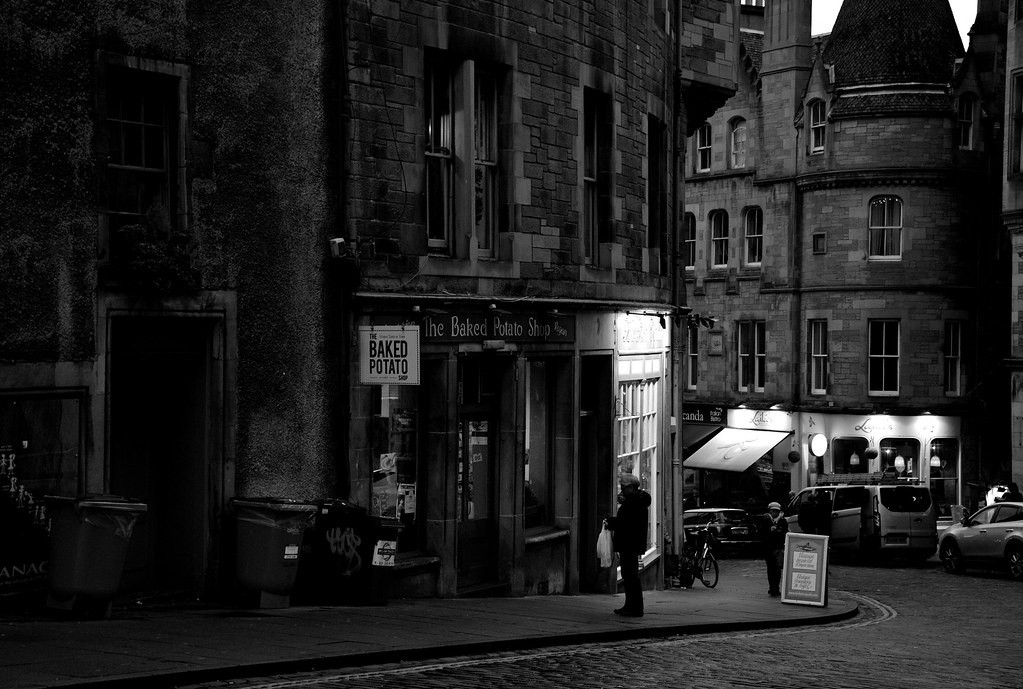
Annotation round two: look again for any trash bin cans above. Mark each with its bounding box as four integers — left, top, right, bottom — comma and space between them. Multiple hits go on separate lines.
230, 495, 318, 607
43, 492, 147, 619
339, 514, 404, 607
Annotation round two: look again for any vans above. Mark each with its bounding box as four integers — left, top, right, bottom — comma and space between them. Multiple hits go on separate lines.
783, 484, 939, 565
684, 508, 758, 555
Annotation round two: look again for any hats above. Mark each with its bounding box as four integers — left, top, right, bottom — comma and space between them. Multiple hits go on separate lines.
768, 503, 781, 511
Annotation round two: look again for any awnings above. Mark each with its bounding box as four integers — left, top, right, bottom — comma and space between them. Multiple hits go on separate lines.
681, 421, 796, 478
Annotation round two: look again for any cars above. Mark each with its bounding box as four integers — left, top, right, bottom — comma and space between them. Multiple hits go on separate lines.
939, 502, 1023, 581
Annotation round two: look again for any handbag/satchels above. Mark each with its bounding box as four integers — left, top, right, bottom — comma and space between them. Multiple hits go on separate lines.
774, 544, 784, 569
596, 519, 614, 568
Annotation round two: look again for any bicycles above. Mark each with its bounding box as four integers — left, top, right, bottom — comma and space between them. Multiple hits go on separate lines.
675, 518, 719, 590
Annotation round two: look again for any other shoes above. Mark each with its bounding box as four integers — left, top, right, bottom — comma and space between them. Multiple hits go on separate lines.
614, 608, 643, 617
768, 589, 781, 596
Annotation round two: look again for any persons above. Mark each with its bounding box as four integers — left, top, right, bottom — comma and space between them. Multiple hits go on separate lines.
604, 478, 652, 617
1003, 480, 1022, 502
797, 488, 833, 533
758, 500, 791, 596
